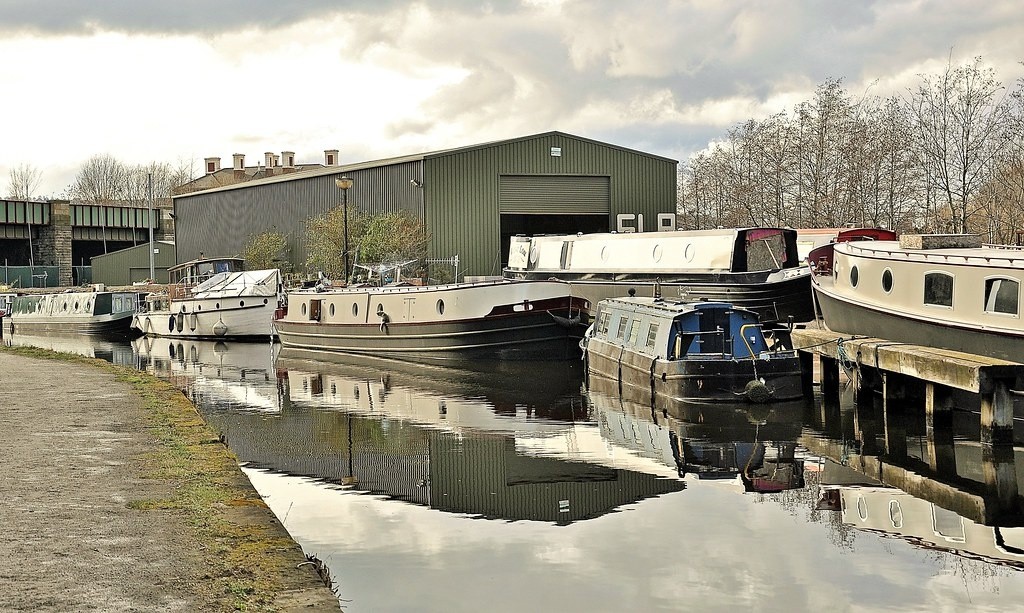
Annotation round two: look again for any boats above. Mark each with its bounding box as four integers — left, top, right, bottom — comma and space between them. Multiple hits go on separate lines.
3, 292, 146, 340
501, 226, 818, 340
809, 234, 1024, 361
579, 297, 806, 402
130, 254, 288, 341
276, 279, 596, 359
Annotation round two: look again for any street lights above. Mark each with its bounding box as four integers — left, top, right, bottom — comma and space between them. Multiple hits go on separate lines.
335, 174, 354, 286
